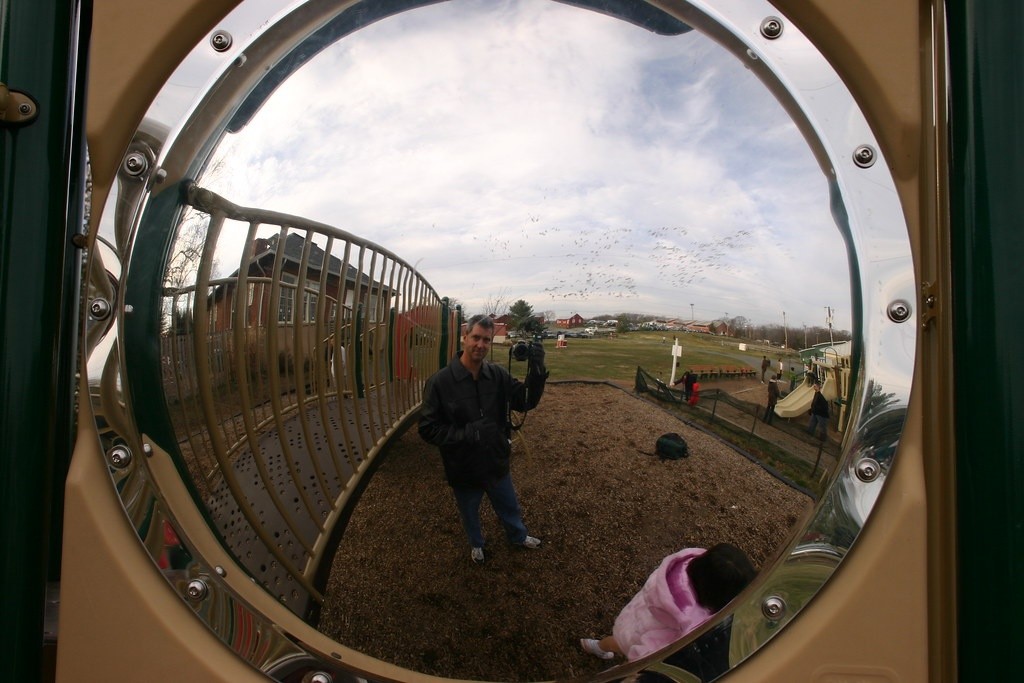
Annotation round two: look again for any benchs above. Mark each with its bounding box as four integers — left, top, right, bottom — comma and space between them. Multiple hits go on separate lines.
718, 365, 742, 378
740, 367, 757, 377
688, 363, 718, 379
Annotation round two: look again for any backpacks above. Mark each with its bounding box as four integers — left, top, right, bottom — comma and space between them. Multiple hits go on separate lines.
656, 433, 689, 460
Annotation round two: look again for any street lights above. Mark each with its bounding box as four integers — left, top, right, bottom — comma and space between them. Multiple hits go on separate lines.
782, 311, 788, 349
803, 324, 808, 350
690, 303, 694, 324
825, 305, 835, 350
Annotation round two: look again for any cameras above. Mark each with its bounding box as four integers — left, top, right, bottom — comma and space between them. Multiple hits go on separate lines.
512, 340, 534, 361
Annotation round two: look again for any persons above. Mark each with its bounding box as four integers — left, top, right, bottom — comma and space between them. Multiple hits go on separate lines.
416, 315, 547, 563
806, 382, 828, 442
330, 337, 348, 399
662, 336, 665, 343
672, 371, 691, 400
760, 356, 784, 385
580, 541, 755, 675
761, 375, 779, 425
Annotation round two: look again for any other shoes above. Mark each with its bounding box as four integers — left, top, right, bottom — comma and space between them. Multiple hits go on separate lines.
580, 638, 614, 659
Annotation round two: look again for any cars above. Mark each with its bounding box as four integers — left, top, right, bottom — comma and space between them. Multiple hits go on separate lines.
542, 319, 683, 342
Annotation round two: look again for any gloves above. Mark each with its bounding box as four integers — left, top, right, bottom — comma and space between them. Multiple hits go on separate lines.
528, 343, 546, 375
469, 416, 496, 441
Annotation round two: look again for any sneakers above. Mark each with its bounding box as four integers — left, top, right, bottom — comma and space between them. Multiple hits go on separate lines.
471, 545, 485, 564
514, 536, 541, 548
